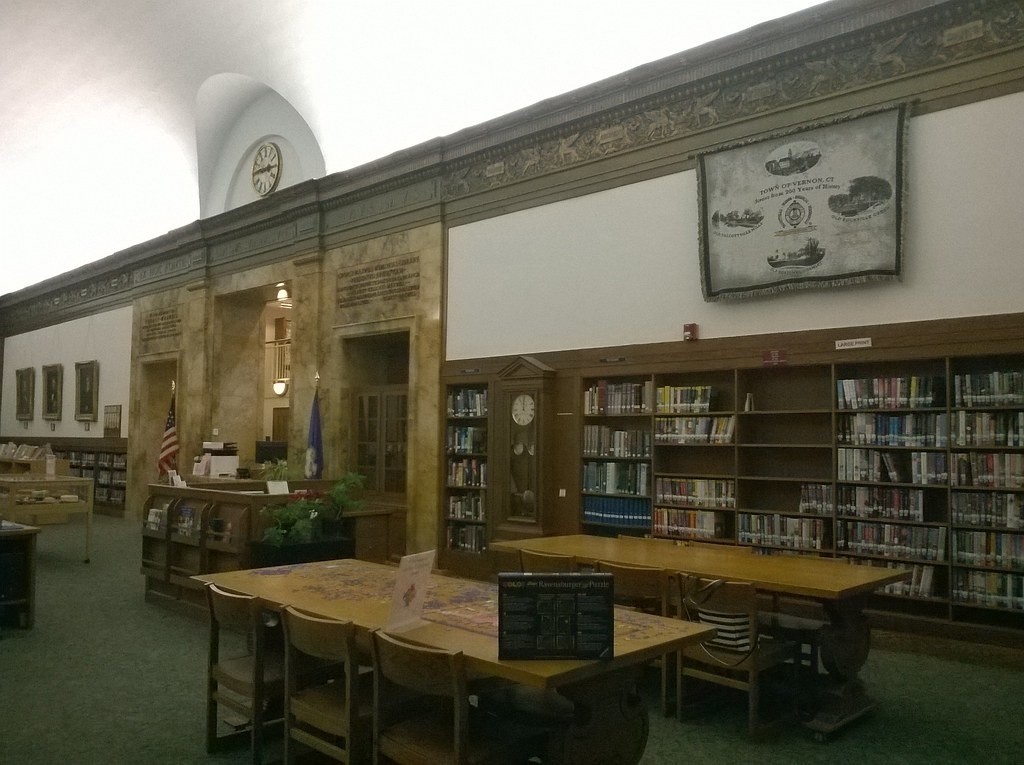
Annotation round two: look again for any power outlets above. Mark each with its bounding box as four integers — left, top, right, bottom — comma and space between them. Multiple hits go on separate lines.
559, 489, 566, 497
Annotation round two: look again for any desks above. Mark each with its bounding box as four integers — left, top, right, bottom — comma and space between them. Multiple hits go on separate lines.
189, 558, 719, 765
0, 474, 94, 564
0, 521, 41, 629
489, 535, 912, 743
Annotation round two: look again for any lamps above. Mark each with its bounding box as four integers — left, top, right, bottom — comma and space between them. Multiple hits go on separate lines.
273, 381, 286, 395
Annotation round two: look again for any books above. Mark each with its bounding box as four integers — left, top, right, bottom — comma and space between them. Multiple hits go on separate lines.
582, 496, 651, 526
654, 507, 726, 539
447, 390, 488, 417
447, 525, 486, 551
656, 385, 712, 413
584, 380, 652, 415
745, 393, 754, 411
448, 457, 487, 487
837, 371, 1024, 611
801, 484, 832, 514
146, 506, 224, 542
738, 514, 826, 549
448, 426, 487, 453
450, 490, 486, 521
0, 442, 125, 504
655, 478, 735, 508
583, 462, 651, 495
583, 424, 651, 458
654, 416, 735, 444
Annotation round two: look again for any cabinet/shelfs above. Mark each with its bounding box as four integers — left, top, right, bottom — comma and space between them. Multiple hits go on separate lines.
440, 376, 490, 580
653, 359, 736, 546
351, 383, 410, 561
0, 438, 128, 524
948, 339, 1024, 629
582, 365, 652, 539
141, 479, 397, 633
833, 345, 948, 619
736, 352, 833, 603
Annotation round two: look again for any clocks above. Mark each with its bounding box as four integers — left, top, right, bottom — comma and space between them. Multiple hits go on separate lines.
252, 143, 279, 196
511, 394, 535, 425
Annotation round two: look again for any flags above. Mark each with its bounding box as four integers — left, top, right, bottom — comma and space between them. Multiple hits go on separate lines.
158, 398, 177, 474
304, 390, 323, 479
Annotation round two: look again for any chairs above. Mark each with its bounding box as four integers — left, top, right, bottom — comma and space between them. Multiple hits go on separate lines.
203, 537, 846, 765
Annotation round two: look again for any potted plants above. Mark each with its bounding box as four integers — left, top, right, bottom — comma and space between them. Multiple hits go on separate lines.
243, 474, 369, 569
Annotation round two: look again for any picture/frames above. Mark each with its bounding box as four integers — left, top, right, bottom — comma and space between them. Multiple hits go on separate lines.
16, 367, 33, 421
42, 364, 63, 421
75, 360, 98, 421
